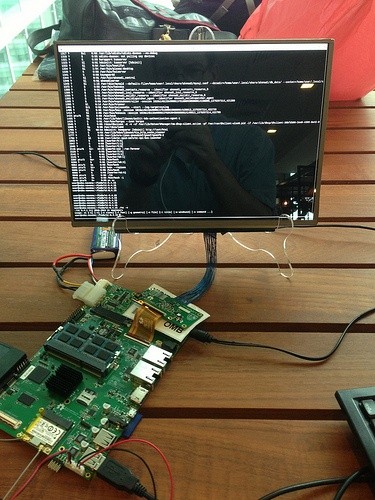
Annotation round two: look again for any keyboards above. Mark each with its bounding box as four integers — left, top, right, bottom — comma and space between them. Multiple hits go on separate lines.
335, 384, 375, 485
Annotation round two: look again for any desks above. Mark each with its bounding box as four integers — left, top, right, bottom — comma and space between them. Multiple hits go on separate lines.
1, 50, 374, 500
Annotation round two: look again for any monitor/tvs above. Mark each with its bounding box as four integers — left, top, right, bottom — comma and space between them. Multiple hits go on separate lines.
54, 40, 334, 227
